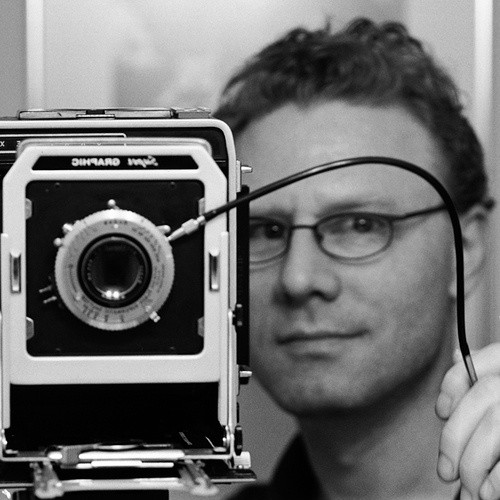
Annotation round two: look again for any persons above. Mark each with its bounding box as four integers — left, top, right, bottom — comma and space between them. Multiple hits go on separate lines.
209, 15, 500, 500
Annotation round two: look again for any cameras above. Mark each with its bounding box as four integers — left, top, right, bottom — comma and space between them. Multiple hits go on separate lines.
0, 108, 252, 486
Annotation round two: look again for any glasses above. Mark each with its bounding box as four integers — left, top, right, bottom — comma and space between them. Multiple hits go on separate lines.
249, 204, 451, 266
